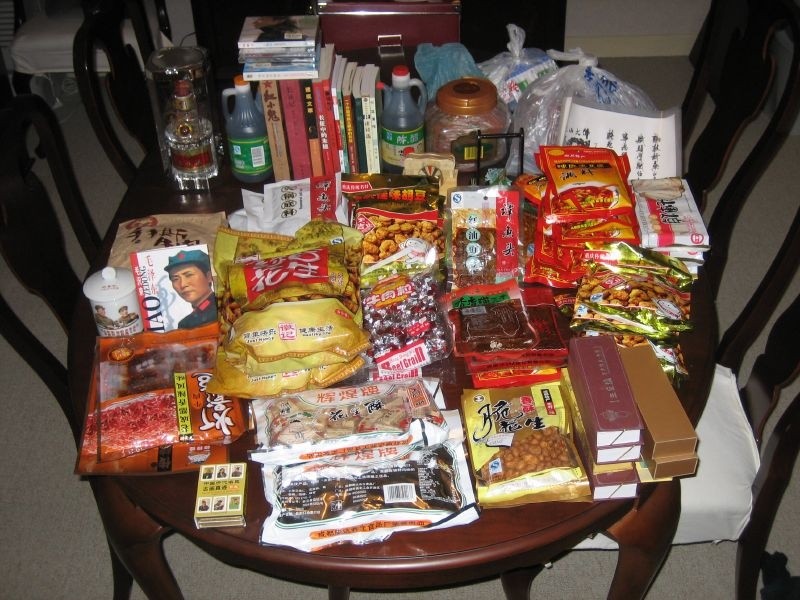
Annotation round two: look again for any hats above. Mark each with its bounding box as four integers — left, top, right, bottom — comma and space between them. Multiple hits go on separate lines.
95, 305, 104, 309
119, 306, 128, 312
164, 250, 210, 270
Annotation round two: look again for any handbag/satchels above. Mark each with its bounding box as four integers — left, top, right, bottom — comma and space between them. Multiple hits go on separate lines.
505, 47, 658, 176
476, 24, 560, 104
414, 42, 485, 103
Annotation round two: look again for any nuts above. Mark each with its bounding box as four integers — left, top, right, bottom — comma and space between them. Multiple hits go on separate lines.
359, 215, 445, 263
482, 427, 579, 481
581, 277, 691, 370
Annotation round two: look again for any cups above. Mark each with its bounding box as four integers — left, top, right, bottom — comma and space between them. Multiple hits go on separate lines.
83, 265, 143, 338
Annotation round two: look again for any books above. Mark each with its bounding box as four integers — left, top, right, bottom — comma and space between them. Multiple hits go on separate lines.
238, 14, 383, 182
130, 244, 219, 334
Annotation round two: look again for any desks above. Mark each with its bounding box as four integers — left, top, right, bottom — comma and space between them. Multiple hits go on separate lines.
74, 150, 716, 600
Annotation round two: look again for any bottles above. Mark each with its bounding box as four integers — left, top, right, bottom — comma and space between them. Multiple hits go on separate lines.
165, 79, 218, 182
222, 74, 274, 183
377, 65, 428, 175
426, 77, 508, 170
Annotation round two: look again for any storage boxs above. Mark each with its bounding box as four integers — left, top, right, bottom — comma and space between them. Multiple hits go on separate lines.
558, 331, 700, 503
316, 0, 460, 60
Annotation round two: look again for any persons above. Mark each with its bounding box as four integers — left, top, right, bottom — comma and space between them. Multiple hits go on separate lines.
95, 305, 140, 328
164, 250, 218, 329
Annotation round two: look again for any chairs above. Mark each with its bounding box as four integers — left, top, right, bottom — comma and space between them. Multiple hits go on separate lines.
500, 203, 800, 600
73, 3, 178, 189
658, 1, 799, 289
0, 69, 133, 600
